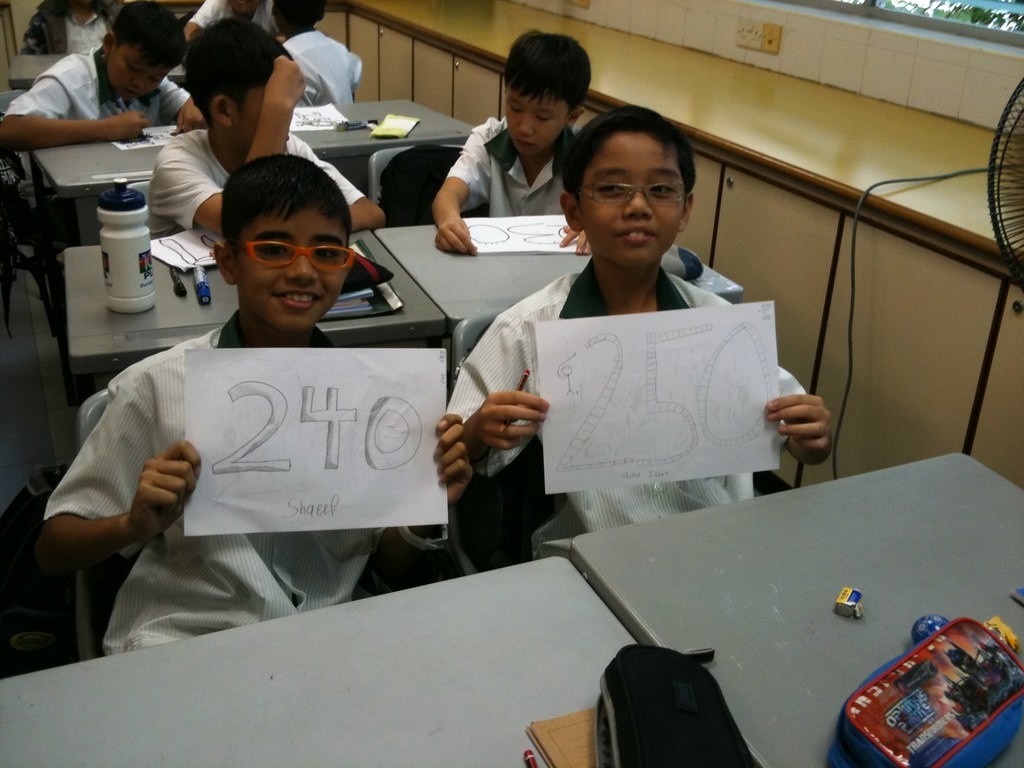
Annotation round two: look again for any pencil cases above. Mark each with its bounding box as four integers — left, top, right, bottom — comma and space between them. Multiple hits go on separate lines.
339, 253, 393, 294
597, 644, 760, 767
824, 617, 1024, 768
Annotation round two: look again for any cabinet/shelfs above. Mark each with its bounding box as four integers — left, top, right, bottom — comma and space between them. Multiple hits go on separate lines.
314, 11, 1024, 492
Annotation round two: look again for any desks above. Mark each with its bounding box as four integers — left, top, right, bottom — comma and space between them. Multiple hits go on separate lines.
0, 52, 1024, 768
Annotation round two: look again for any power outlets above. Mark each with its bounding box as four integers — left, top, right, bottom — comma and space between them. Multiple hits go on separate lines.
735, 17, 783, 55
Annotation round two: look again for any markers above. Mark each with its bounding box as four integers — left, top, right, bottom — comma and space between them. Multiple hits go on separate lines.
193, 265, 212, 307
335, 118, 377, 132
523, 749, 538, 768
169, 265, 187, 297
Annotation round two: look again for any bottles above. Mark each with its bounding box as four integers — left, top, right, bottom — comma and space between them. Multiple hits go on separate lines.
96, 177, 158, 314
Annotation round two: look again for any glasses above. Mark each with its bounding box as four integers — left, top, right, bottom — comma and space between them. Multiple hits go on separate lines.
575, 178, 685, 204
243, 240, 355, 272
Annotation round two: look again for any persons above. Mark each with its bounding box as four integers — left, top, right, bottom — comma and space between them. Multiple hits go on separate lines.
0, 0, 210, 201
271, 0, 363, 105
184, 0, 287, 44
431, 30, 592, 255
37, 154, 475, 657
446, 104, 833, 560
20, 0, 123, 56
149, 17, 386, 241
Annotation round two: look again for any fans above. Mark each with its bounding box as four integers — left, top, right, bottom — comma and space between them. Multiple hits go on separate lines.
986, 76, 1024, 289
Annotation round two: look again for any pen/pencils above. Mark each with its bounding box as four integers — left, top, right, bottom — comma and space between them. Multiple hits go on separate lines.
117, 97, 128, 114
